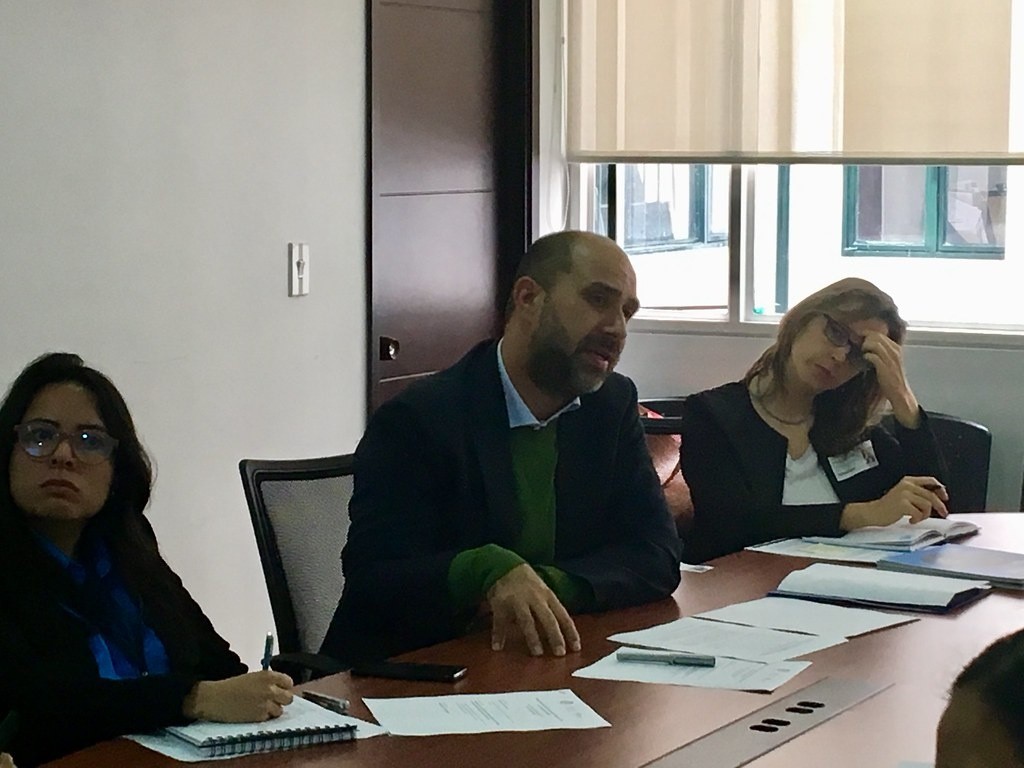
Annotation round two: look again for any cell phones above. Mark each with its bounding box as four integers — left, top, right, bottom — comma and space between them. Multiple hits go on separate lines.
351, 663, 467, 683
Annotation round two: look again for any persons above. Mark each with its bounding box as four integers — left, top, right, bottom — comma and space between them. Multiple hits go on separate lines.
0, 352, 295, 764
680, 279, 949, 564
934, 628, 1024, 768
306, 231, 680, 678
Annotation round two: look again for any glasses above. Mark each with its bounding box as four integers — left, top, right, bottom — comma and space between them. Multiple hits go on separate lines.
12, 417, 118, 467
821, 312, 875, 373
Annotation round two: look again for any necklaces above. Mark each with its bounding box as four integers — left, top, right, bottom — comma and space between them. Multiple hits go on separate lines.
757, 374, 814, 425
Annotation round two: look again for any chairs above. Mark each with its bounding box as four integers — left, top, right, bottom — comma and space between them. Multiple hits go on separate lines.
239, 453, 358, 687
880, 409, 992, 519
637, 397, 695, 542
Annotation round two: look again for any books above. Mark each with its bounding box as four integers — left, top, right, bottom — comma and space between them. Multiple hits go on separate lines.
167, 692, 358, 759
800, 514, 1024, 589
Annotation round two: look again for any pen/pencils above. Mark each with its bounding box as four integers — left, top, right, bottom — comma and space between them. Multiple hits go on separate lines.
263, 630, 274, 670
616, 651, 716, 668
302, 689, 350, 711
929, 482, 942, 492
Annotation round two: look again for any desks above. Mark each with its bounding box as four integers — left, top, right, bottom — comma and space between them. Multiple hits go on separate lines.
42, 512, 1024, 767
